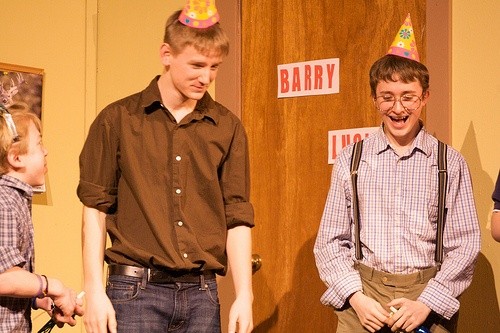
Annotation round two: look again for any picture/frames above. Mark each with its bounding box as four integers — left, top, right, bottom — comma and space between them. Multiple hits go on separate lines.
0, 64, 46, 193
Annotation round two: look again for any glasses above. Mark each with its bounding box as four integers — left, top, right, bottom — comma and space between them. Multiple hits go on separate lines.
373, 93, 424, 109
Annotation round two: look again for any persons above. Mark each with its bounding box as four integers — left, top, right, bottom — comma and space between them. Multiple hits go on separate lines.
313, 13, 481, 333
76, 0, 255, 333
0, 113, 84, 333
491, 169, 500, 242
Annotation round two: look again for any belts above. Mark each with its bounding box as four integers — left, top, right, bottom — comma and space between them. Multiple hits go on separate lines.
108, 264, 216, 282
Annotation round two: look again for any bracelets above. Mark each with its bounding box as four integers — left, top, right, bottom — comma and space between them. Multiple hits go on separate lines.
32, 298, 38, 310
36, 275, 49, 299
